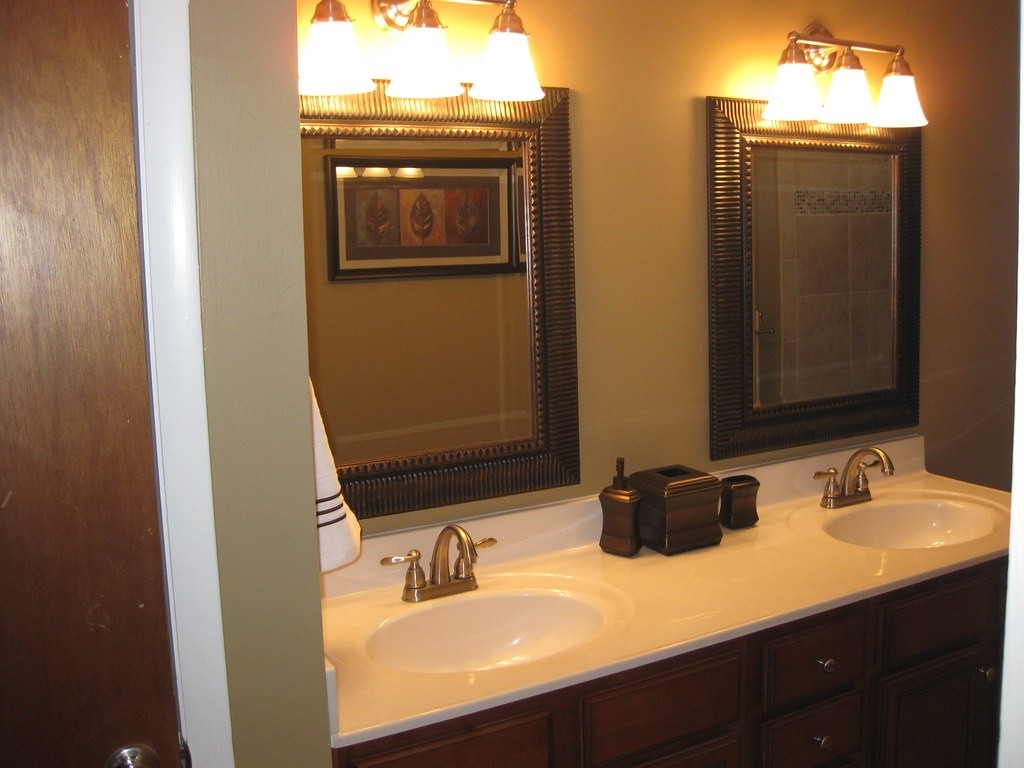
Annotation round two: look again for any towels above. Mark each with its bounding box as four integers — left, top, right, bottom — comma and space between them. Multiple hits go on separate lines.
308, 376, 361, 574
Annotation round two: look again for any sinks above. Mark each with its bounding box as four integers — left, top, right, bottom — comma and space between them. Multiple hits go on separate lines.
354, 583, 615, 678
816, 492, 999, 554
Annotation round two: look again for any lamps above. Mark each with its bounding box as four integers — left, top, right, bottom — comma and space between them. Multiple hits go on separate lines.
765, 22, 931, 130
298, 0, 544, 103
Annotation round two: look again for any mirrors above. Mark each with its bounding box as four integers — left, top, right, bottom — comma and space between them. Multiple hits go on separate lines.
298, 79, 582, 522
702, 97, 922, 462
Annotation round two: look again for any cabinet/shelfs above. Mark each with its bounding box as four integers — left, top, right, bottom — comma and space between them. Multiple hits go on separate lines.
340, 560, 1009, 768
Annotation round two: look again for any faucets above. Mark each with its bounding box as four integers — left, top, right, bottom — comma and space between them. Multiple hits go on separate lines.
429, 523, 479, 586
841, 447, 896, 497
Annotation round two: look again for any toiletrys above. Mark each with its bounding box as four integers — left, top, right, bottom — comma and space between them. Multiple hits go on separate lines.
596, 457, 643, 560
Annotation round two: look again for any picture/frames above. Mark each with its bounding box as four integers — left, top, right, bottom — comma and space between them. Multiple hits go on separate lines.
512, 160, 528, 272
324, 154, 513, 281
